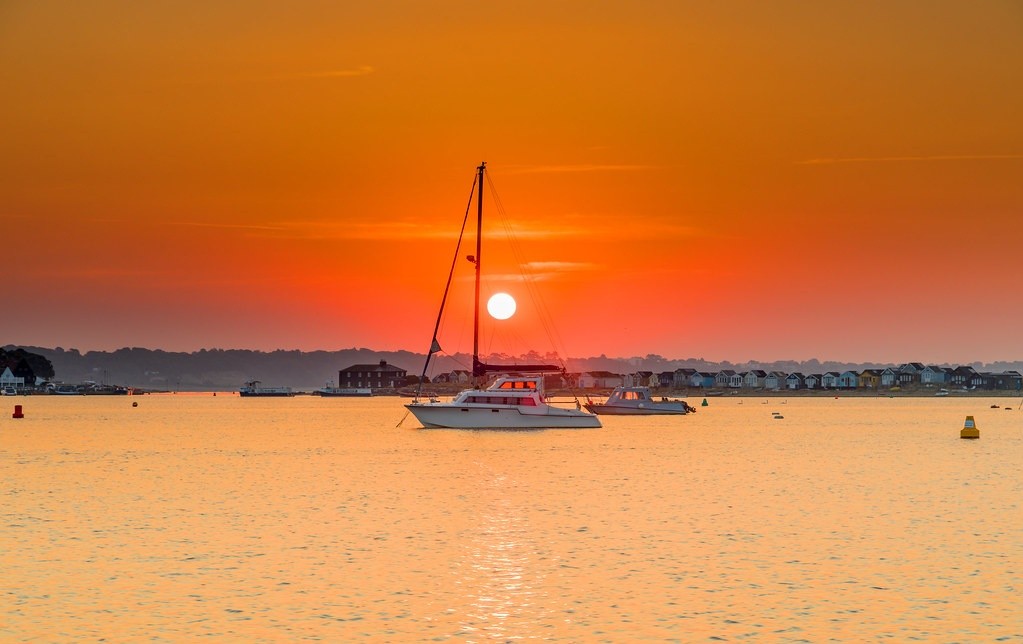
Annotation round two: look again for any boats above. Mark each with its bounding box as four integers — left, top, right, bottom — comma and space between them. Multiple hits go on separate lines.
582, 386, 697, 416
313, 380, 373, 397
1, 381, 145, 395
240, 379, 295, 397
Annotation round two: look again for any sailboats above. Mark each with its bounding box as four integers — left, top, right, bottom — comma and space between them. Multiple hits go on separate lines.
407, 161, 604, 431
